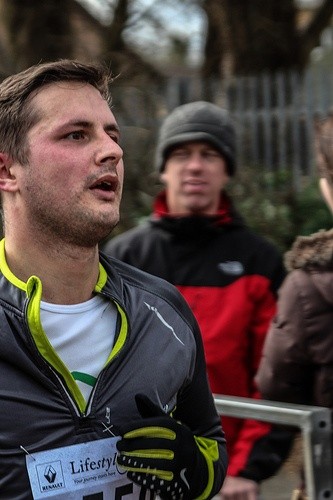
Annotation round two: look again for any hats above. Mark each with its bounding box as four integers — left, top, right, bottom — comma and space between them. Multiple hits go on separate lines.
156, 101, 239, 178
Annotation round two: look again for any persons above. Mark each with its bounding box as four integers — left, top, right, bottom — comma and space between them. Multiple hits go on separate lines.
256, 109, 332, 500
104, 101, 301, 499
0, 58, 225, 500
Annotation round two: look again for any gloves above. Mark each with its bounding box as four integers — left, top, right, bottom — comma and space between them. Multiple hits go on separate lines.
115, 393, 219, 500
